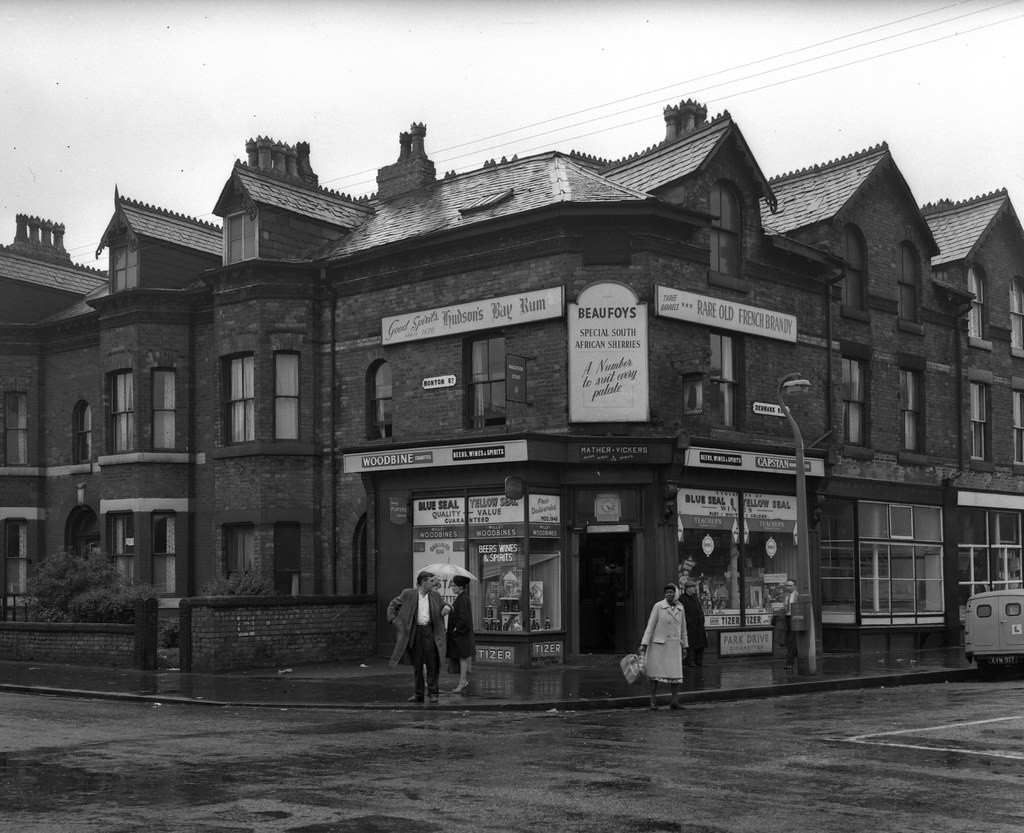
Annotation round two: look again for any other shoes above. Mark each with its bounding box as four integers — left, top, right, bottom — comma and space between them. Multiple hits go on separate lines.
650, 702, 658, 710
408, 696, 424, 703
430, 697, 438, 703
670, 702, 685, 710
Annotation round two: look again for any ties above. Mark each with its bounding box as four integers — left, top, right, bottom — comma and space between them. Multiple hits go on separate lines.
786, 595, 790, 613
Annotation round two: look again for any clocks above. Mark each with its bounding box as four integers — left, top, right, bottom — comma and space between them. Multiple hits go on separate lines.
594, 493, 621, 522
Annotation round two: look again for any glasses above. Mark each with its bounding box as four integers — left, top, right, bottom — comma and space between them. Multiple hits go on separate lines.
785, 585, 795, 587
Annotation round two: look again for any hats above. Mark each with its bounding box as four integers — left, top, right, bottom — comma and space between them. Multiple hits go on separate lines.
683, 580, 697, 587
453, 575, 470, 586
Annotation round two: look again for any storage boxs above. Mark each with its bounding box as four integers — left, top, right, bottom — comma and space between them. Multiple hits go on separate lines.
529, 581, 543, 605
499, 564, 522, 597
484, 581, 503, 606
502, 611, 523, 632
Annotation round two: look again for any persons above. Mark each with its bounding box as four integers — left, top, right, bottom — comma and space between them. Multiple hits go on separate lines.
678, 580, 707, 667
775, 579, 798, 669
639, 583, 689, 710
446, 576, 476, 693
387, 571, 454, 703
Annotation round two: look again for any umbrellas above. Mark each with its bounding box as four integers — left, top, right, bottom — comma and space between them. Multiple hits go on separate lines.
414, 557, 479, 601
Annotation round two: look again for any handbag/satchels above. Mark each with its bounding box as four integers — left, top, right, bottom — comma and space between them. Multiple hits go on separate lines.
447, 658, 460, 675
619, 650, 646, 685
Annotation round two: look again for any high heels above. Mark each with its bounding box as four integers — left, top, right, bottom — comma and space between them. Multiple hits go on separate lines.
448, 680, 470, 694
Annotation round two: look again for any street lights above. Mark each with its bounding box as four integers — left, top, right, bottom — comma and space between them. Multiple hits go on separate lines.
778, 372, 818, 676
